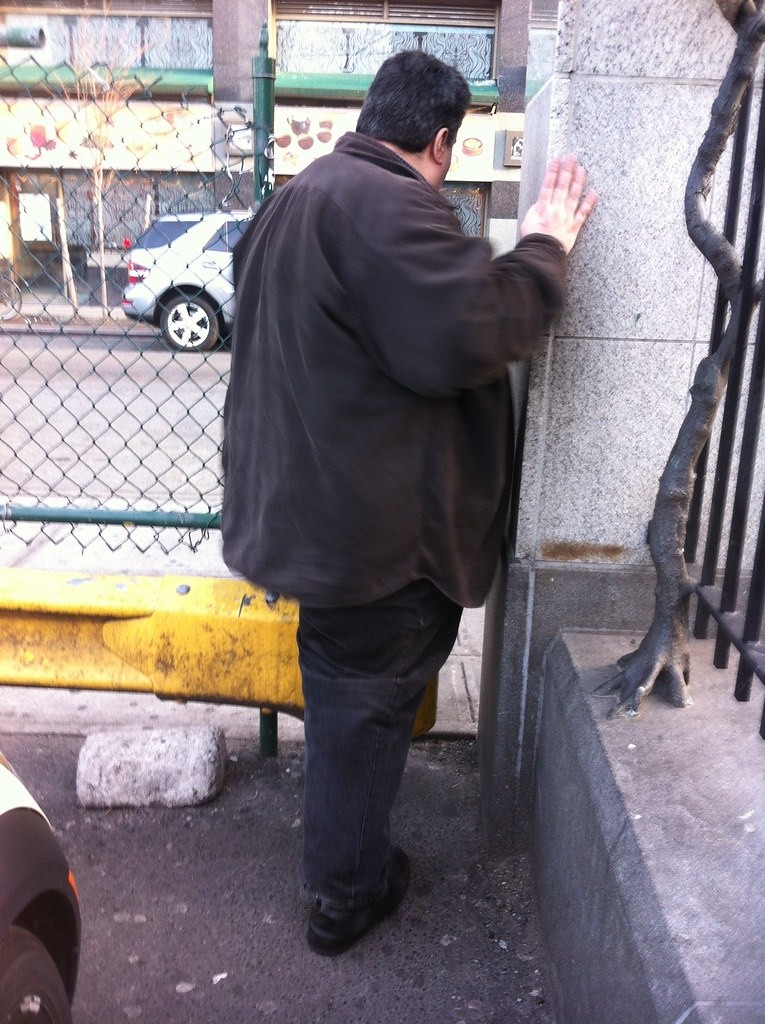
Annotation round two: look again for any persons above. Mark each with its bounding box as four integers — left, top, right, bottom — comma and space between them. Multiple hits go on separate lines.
217, 46, 598, 959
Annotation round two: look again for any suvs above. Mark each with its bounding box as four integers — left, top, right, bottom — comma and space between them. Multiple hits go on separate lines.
122, 211, 255, 354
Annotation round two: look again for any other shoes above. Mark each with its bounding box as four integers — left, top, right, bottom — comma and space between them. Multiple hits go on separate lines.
307, 847, 411, 957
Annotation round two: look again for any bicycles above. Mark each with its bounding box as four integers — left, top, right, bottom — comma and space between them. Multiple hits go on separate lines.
0, 260, 22, 321
